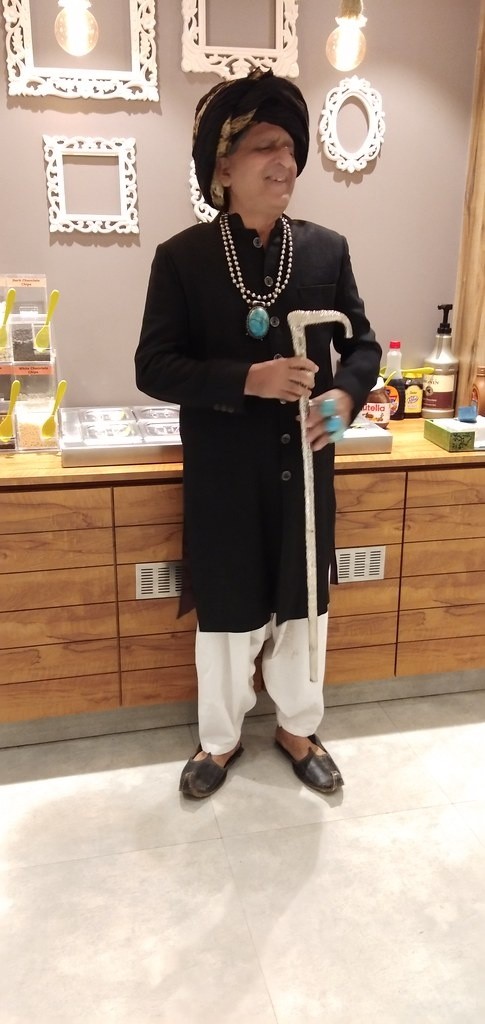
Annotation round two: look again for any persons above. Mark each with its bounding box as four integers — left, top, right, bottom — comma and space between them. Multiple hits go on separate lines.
132, 73, 385, 801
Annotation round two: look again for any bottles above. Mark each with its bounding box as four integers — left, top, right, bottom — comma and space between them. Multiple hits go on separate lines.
383, 340, 405, 421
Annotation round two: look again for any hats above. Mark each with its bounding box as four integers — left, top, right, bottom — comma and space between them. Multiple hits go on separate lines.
192, 67, 309, 212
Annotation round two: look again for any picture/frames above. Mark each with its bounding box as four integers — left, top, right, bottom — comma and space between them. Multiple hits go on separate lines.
2, 0, 159, 104
189, 158, 220, 222
319, 75, 386, 176
179, 0, 301, 82
42, 134, 140, 235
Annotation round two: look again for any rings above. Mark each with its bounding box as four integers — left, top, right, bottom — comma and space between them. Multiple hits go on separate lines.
326, 415, 342, 432
329, 429, 343, 443
321, 397, 336, 417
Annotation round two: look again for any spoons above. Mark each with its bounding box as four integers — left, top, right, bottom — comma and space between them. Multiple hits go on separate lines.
0, 288, 17, 347
41, 380, 68, 439
0, 380, 20, 442
36, 290, 60, 351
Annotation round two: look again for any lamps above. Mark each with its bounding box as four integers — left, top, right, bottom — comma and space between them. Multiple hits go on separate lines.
325, 0, 368, 72
53, 0, 99, 56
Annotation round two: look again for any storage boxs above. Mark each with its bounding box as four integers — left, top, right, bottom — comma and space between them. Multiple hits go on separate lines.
0, 273, 59, 453
335, 422, 393, 455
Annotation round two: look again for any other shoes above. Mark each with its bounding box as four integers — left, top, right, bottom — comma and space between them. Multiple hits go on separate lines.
179, 741, 244, 797
275, 734, 344, 792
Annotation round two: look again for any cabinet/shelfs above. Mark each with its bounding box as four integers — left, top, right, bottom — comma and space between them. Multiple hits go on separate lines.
0, 418, 485, 725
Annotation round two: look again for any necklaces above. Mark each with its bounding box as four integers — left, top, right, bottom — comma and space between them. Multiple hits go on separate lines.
218, 212, 293, 342
